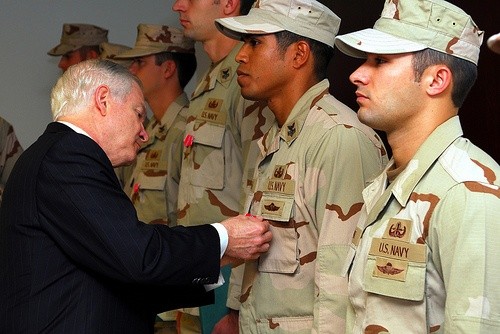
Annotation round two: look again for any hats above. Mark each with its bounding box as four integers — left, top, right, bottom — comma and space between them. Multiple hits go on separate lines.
114, 22, 195, 59
46, 23, 109, 56
334, 0, 484, 66
213, 0, 342, 50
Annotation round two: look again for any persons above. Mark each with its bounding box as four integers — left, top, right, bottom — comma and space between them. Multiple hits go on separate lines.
0, 58, 273, 334
0, 0, 500, 334
